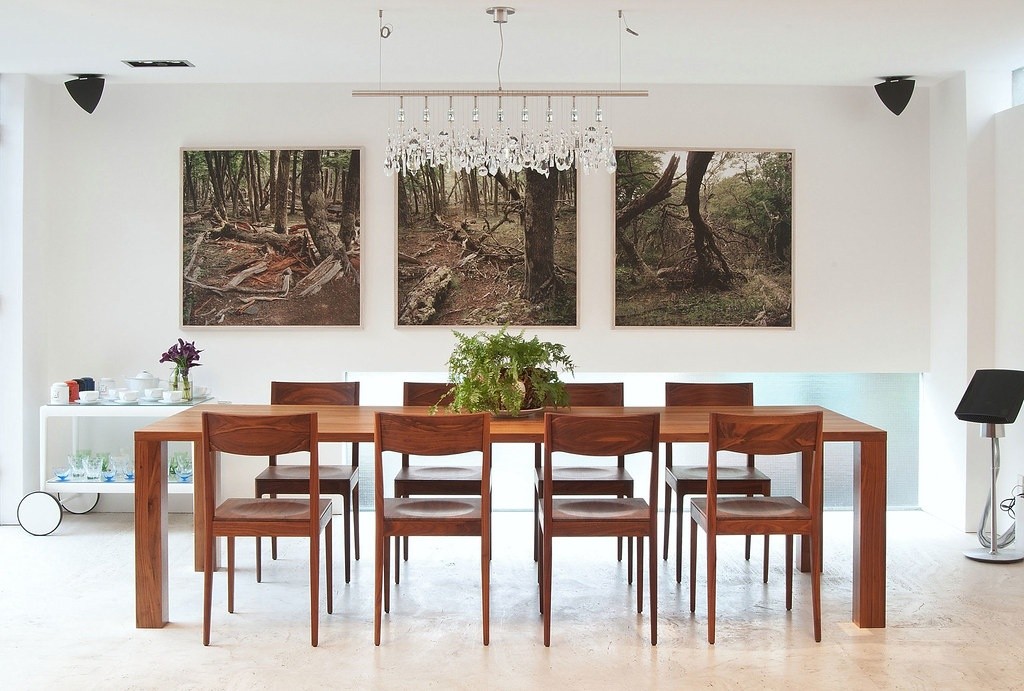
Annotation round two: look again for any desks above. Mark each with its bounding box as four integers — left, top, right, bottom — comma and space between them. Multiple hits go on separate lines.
133, 401, 888, 631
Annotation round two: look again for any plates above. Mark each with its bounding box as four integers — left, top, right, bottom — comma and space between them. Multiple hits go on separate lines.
75, 398, 188, 404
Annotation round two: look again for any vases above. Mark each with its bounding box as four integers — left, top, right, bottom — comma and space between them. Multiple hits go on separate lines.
178, 367, 193, 401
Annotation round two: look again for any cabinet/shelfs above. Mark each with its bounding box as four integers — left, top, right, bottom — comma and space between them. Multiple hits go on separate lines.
18, 397, 220, 536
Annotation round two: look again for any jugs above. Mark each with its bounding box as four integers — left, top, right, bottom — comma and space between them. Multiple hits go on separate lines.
131, 371, 159, 392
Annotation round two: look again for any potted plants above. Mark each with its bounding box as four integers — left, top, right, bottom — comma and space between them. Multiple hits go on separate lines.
431, 324, 579, 415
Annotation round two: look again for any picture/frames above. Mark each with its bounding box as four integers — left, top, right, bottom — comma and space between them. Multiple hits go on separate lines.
610, 145, 795, 332
179, 147, 367, 329
395, 162, 582, 329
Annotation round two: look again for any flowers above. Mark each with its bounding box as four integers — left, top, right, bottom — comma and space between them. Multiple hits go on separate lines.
159, 338, 205, 400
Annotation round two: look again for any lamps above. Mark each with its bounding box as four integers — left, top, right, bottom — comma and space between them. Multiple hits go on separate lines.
349, 6, 649, 178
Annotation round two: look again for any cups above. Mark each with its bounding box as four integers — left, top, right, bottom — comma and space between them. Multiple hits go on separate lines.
65, 378, 181, 401
51, 383, 69, 404
54, 455, 192, 483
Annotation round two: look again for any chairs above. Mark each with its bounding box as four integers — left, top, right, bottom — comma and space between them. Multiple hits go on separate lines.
201, 381, 826, 649
